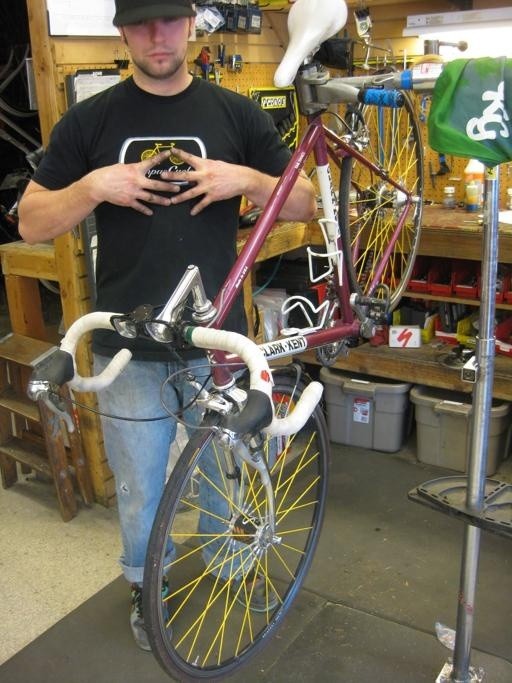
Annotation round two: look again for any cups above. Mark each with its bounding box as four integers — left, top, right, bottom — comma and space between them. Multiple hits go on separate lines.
465, 170, 485, 211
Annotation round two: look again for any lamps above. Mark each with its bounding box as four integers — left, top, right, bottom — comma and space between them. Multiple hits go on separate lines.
423, 39, 468, 59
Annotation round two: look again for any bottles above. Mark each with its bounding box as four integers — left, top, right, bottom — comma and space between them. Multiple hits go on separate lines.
442, 186, 455, 208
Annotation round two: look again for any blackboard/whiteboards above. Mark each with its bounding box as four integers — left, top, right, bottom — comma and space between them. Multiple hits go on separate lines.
46, 0, 121, 37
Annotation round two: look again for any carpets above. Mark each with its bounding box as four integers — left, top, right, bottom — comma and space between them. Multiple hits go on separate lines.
2, 441, 512, 683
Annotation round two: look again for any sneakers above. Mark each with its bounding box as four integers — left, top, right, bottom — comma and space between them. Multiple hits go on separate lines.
200, 562, 282, 614
126, 573, 176, 651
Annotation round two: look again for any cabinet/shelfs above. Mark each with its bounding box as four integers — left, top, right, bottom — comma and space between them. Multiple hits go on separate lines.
230, 204, 512, 401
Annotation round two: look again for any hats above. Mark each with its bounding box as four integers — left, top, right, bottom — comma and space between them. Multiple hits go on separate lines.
108, 0, 203, 28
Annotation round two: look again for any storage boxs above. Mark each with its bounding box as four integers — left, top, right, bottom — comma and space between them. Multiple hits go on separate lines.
317, 365, 416, 453
409, 384, 510, 475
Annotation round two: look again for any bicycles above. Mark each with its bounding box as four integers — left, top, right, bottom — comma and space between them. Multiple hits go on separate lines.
0, 30, 61, 295
21, 0, 427, 682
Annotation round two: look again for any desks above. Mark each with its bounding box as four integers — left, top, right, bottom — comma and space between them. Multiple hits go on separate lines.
5, 241, 64, 435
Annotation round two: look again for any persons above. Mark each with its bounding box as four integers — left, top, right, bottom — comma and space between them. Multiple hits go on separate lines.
17, 0, 317, 653
